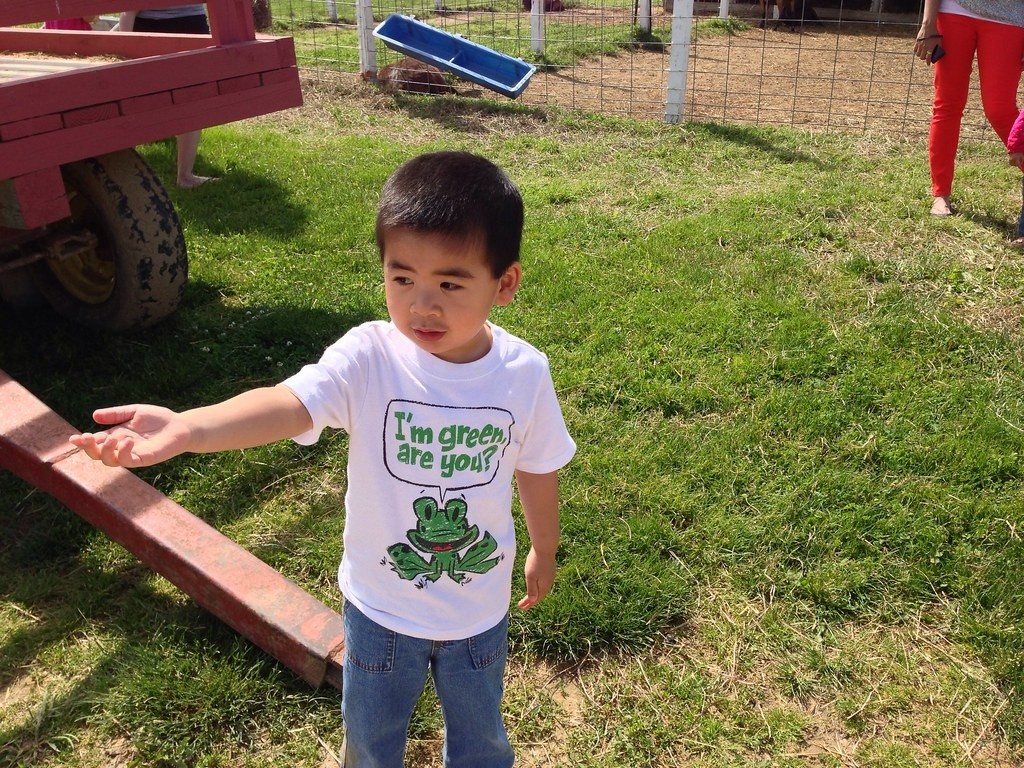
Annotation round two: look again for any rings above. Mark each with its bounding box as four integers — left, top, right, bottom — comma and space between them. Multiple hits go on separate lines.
927, 51, 932, 55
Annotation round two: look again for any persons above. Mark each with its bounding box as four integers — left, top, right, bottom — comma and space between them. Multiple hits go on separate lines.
913, 0, 1024, 246
69, 152, 577, 768
44, 0, 221, 189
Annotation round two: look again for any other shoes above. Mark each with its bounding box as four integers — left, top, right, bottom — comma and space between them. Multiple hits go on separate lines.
930, 195, 952, 215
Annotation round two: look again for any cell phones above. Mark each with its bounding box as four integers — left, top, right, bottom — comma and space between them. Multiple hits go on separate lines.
930, 45, 945, 63
